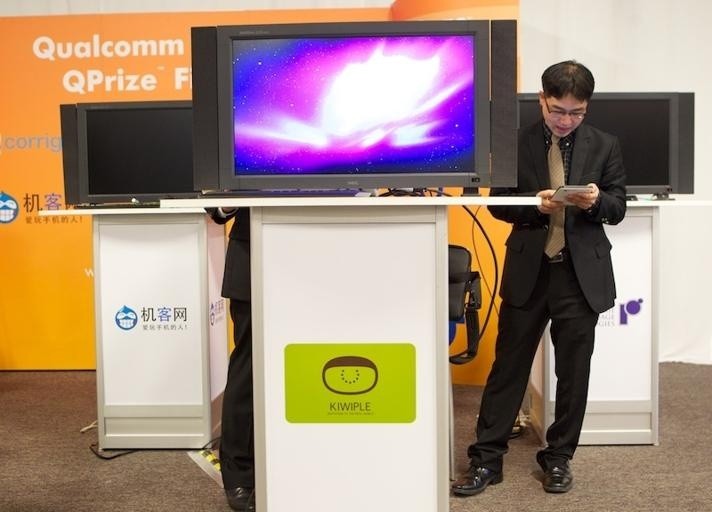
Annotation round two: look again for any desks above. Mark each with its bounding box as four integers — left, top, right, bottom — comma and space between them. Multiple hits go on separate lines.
158, 199, 542, 510
528, 198, 670, 448
38, 199, 230, 450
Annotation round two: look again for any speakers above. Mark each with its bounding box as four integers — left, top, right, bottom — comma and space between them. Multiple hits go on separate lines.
490, 19, 520, 190
680, 92, 694, 195
58, 104, 79, 208
189, 25, 219, 192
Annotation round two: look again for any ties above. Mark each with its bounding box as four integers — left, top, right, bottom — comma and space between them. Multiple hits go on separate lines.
544, 133, 566, 259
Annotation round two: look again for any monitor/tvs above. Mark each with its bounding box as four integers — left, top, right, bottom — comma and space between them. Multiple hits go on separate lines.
78, 100, 193, 205
517, 92, 680, 193
219, 22, 489, 191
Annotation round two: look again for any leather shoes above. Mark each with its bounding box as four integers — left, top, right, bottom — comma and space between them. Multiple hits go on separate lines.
452, 465, 503, 495
543, 466, 574, 493
224, 484, 255, 512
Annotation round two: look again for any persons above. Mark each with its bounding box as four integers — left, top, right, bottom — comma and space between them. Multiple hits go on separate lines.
450, 60, 628, 495
202, 207, 255, 512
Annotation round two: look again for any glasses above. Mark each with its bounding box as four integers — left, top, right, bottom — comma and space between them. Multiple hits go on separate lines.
544, 96, 588, 119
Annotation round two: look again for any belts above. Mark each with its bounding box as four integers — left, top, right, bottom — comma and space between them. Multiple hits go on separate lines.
545, 250, 570, 264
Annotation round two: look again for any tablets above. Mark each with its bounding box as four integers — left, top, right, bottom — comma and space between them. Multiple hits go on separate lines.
548, 184, 594, 208
